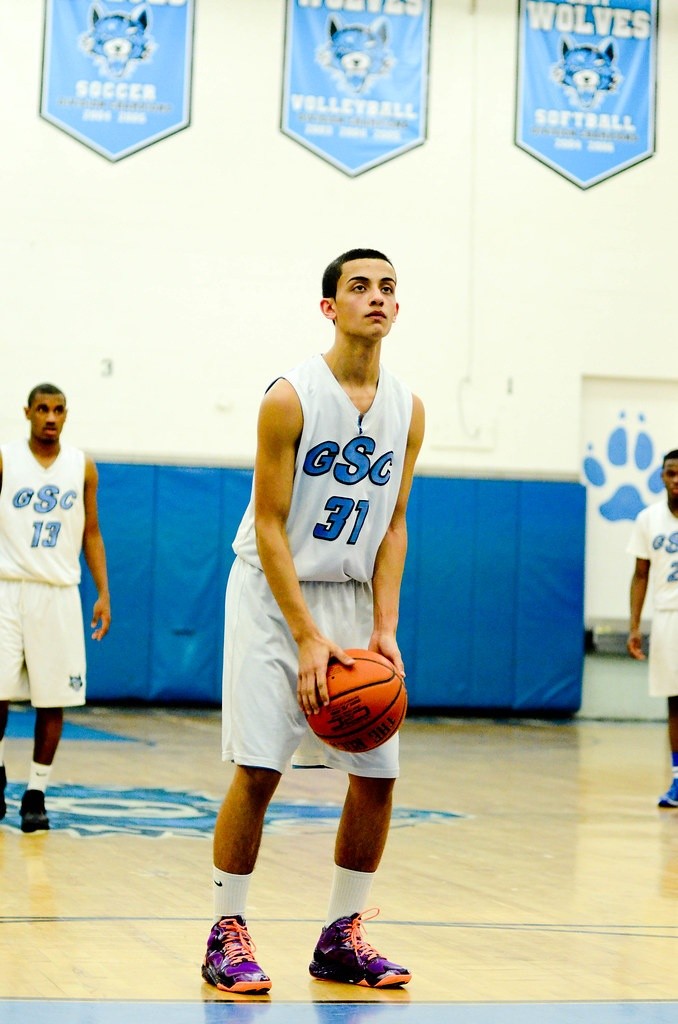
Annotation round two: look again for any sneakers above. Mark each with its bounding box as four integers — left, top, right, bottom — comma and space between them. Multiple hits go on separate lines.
308, 908, 412, 987
658, 778, 678, 807
200, 915, 273, 993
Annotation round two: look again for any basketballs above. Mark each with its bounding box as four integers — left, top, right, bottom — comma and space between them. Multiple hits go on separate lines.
300, 649, 407, 754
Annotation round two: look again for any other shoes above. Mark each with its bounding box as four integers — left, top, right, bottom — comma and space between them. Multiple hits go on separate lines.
0, 766, 7, 820
19, 788, 51, 833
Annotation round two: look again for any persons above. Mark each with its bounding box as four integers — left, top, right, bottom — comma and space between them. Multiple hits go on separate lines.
627, 448, 678, 808
0, 384, 111, 833
204, 249, 425, 994
